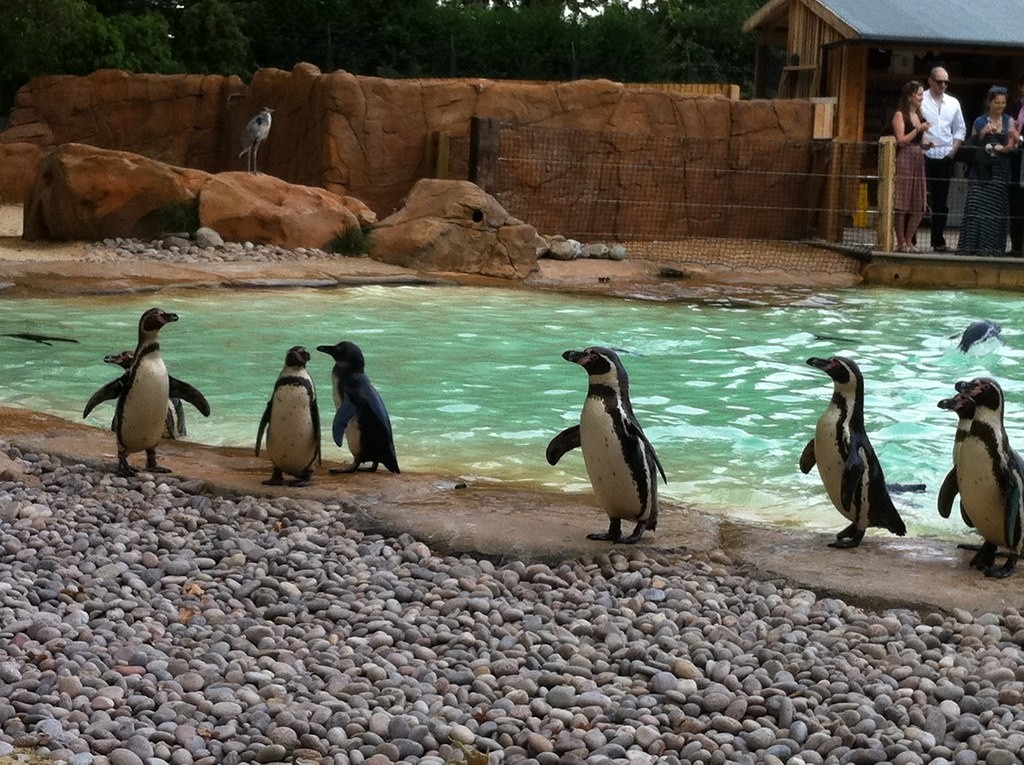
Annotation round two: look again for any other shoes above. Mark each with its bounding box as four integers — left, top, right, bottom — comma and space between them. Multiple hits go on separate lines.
934, 246, 955, 254
894, 245, 921, 253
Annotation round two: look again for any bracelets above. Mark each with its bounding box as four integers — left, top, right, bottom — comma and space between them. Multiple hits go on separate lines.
916, 127, 921, 132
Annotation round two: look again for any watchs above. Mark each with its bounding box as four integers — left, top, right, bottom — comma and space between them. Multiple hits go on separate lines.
1019, 134, 1023, 143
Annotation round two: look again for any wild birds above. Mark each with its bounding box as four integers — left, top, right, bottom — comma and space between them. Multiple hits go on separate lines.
238, 105, 276, 177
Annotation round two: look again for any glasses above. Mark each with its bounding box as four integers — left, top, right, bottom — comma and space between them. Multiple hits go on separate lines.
932, 78, 951, 86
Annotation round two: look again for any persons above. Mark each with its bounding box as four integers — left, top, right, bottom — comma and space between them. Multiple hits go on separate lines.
900, 64, 967, 254
888, 80, 938, 253
954, 85, 1019, 258
1005, 86, 1024, 259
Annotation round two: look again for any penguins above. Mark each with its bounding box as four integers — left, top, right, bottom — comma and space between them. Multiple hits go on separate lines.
316, 341, 401, 475
81, 305, 212, 479
543, 347, 668, 545
253, 345, 322, 488
795, 355, 907, 550
936, 376, 1024, 579
948, 318, 1006, 356
